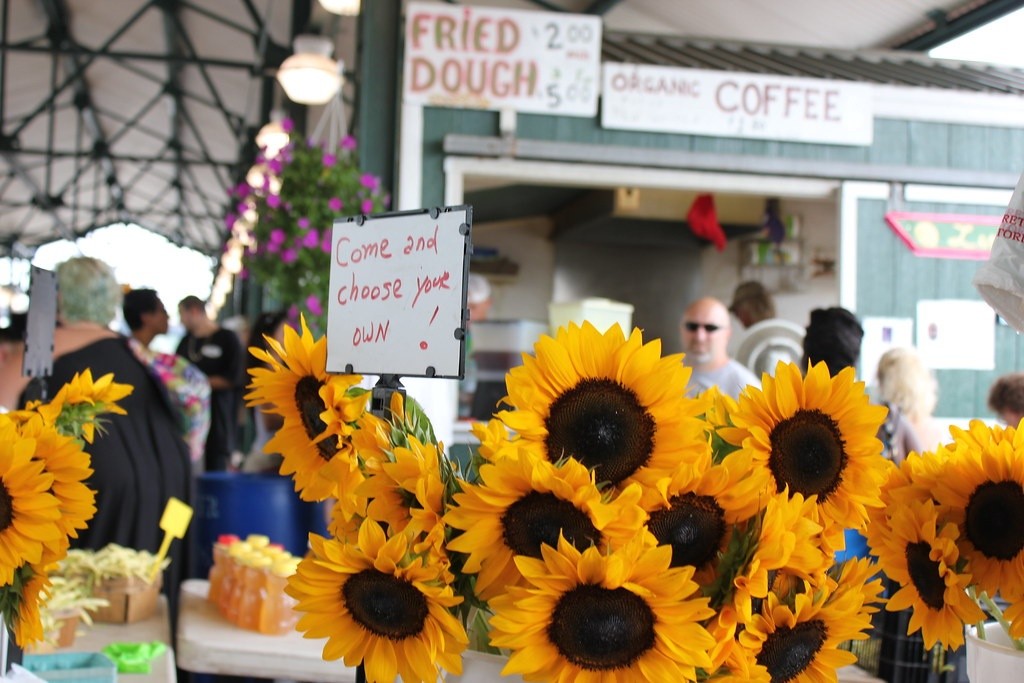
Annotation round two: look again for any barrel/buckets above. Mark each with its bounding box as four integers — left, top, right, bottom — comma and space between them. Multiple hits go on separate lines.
193, 473, 306, 580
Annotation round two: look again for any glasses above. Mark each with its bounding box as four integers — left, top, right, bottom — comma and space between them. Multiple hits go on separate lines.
684, 321, 718, 332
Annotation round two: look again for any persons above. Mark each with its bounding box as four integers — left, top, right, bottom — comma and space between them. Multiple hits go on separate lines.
682, 297, 763, 401
990, 373, 1024, 429
798, 306, 933, 574
124, 288, 243, 471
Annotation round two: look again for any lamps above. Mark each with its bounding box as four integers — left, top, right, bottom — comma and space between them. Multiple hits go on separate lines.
203, 36, 346, 324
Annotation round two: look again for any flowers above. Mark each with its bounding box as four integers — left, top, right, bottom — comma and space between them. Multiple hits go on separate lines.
0, 367, 135, 675
225, 116, 390, 333
242, 311, 1024, 683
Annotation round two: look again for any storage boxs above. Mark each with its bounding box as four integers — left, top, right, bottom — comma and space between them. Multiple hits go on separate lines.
22, 651, 118, 683
86, 572, 161, 624
